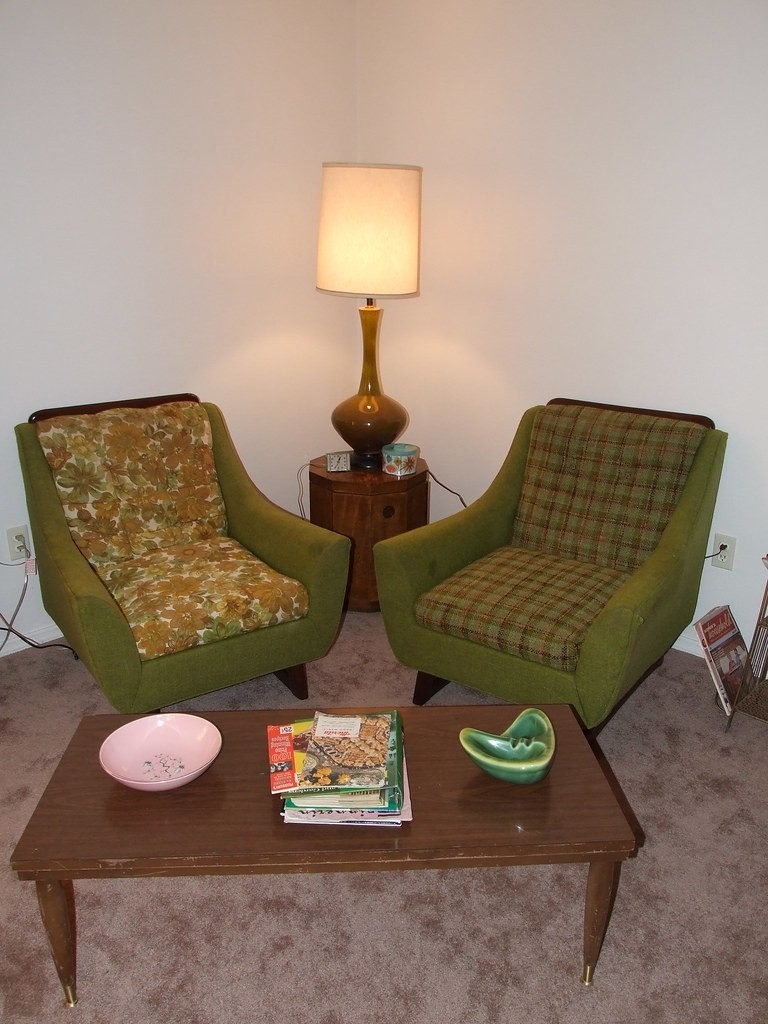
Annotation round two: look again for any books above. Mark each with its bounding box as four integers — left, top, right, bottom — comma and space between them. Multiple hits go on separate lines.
694, 605, 755, 717
267, 712, 412, 828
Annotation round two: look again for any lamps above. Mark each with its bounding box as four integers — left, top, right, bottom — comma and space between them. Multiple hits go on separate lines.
312, 160, 424, 473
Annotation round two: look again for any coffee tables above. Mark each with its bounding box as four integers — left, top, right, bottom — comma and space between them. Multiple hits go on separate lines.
9, 704, 637, 1008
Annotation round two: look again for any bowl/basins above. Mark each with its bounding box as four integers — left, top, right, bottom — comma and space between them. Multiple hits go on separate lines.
381, 444, 420, 475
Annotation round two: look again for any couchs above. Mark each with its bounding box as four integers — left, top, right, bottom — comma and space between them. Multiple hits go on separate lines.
14, 392, 352, 720
371, 396, 729, 734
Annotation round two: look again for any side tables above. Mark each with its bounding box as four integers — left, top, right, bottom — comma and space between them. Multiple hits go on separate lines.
309, 450, 430, 613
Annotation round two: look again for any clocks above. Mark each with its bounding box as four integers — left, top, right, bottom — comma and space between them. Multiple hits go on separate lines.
325, 452, 351, 473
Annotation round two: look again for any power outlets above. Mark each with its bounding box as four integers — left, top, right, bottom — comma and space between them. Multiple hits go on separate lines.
6, 523, 32, 561
711, 532, 736, 572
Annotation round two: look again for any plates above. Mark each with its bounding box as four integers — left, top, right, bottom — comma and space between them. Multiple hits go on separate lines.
100, 713, 222, 791
